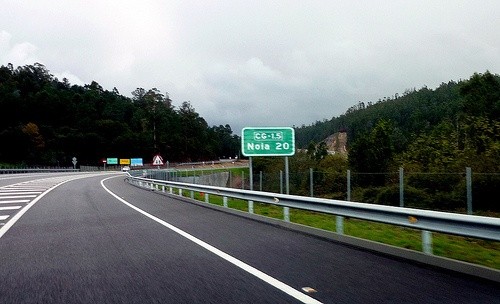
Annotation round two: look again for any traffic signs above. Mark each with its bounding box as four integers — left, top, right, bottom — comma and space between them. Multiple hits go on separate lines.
241, 127, 295, 156
